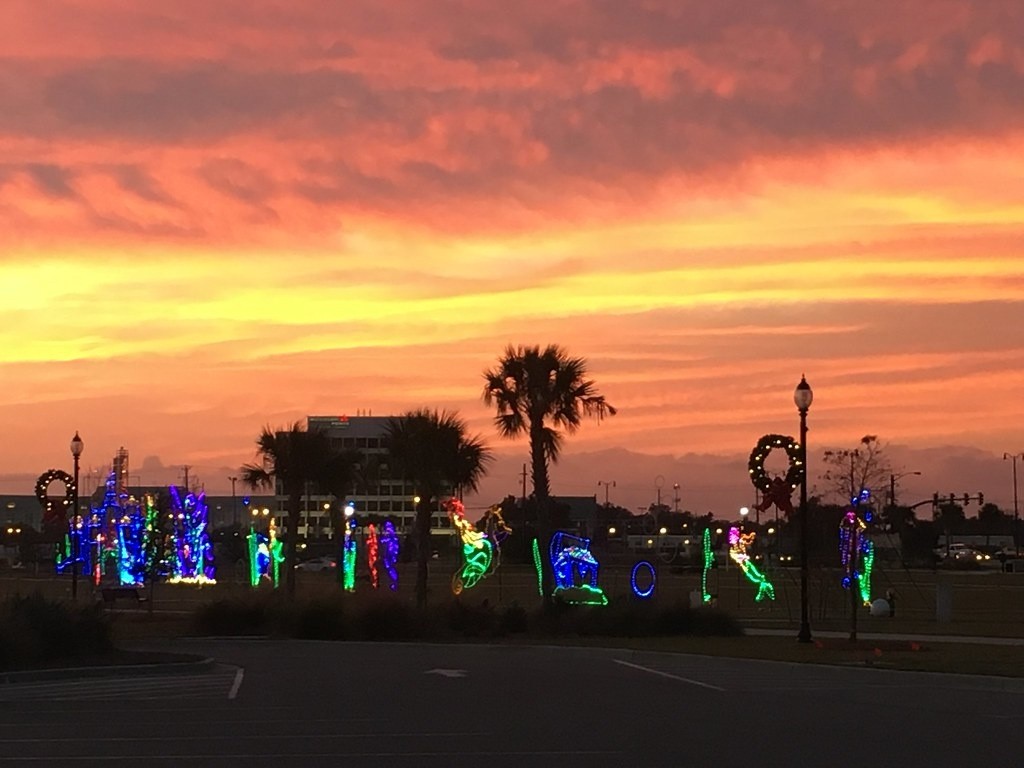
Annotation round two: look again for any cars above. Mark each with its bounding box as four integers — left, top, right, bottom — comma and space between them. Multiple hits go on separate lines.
932, 540, 1022, 563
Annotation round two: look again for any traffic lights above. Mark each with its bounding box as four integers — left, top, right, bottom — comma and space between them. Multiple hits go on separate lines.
964, 493, 970, 506
979, 493, 984, 505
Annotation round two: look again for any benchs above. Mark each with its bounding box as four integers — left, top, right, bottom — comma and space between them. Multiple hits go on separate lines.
101, 588, 150, 618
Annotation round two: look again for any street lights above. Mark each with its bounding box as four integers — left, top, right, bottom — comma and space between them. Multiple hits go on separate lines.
891, 472, 921, 505
1003, 452, 1023, 557
793, 374, 814, 642
70, 430, 84, 600
229, 476, 237, 525
519, 464, 529, 541
597, 480, 616, 562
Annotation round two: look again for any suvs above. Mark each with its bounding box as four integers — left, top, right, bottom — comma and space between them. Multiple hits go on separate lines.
652, 542, 683, 565
